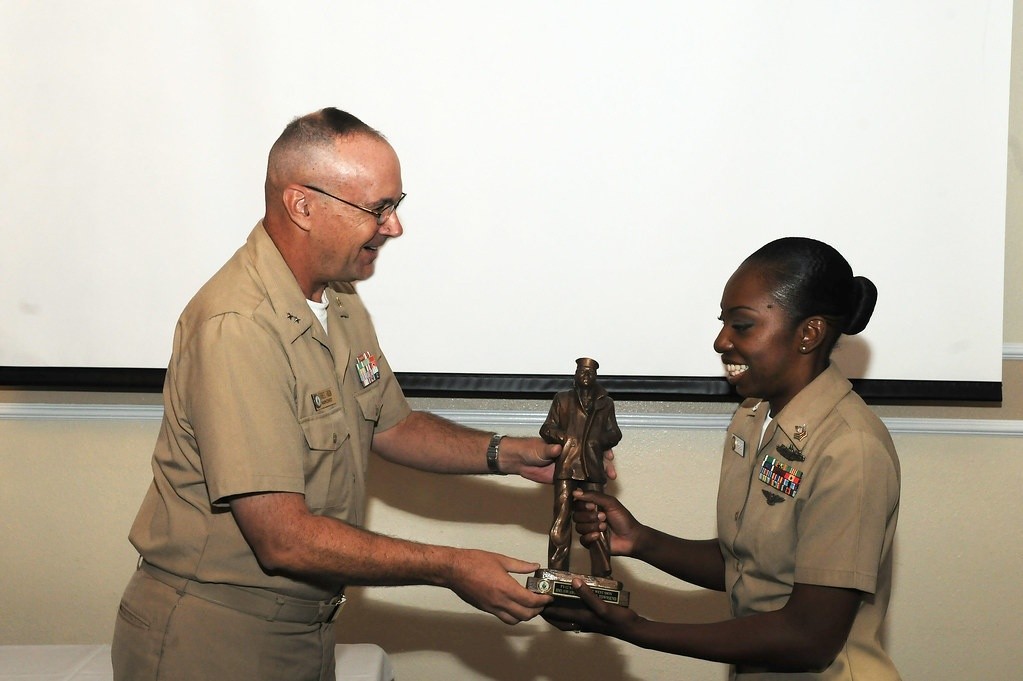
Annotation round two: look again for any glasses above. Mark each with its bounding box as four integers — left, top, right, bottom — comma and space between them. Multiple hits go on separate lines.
301, 184, 408, 225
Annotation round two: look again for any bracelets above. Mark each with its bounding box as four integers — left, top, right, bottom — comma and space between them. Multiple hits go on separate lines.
486, 433, 509, 476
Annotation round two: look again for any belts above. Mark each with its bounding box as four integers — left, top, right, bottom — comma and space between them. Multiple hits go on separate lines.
140, 560, 346, 623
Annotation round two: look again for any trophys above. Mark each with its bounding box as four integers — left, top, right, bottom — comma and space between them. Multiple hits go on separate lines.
524, 357, 631, 609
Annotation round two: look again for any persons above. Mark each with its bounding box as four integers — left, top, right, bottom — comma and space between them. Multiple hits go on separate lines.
538, 235, 904, 681
111, 106, 618, 681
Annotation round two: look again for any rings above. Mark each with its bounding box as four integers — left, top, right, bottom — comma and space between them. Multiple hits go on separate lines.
571, 623, 580, 634
